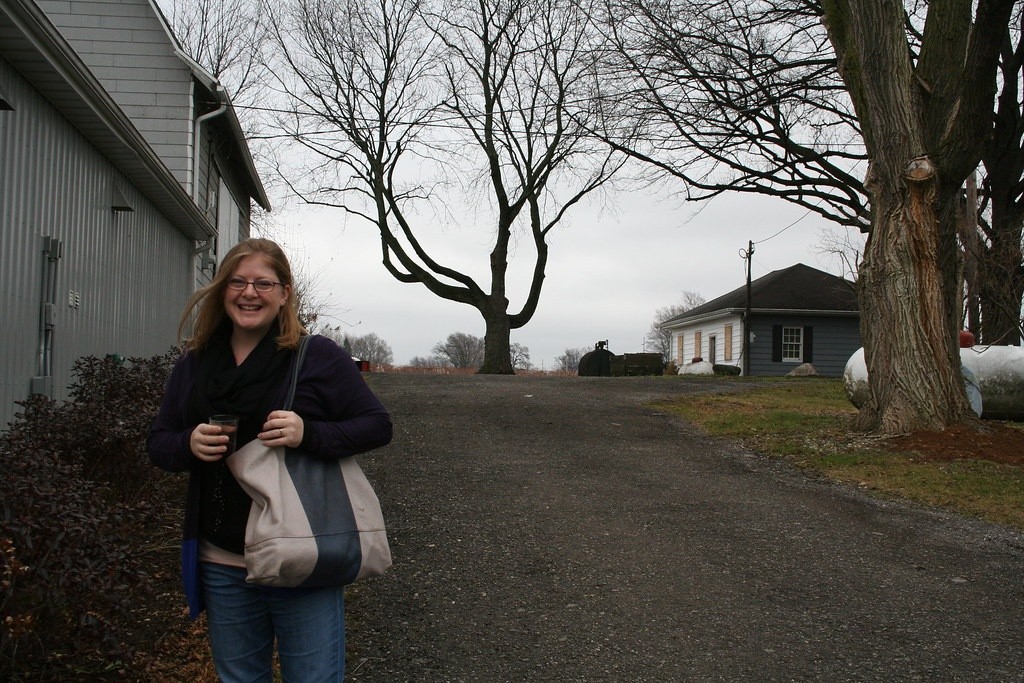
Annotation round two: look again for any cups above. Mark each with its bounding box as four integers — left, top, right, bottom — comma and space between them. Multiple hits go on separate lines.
207, 416, 238, 459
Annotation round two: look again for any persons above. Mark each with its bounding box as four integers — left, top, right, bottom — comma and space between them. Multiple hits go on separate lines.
143, 239, 396, 683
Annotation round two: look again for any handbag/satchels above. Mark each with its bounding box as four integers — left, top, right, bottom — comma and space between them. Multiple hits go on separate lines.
225, 333, 394, 590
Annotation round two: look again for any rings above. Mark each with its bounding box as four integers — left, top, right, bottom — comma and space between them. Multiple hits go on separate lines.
280, 429, 283, 437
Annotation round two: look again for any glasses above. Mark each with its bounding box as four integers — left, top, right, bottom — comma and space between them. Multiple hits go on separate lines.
225, 275, 288, 292
